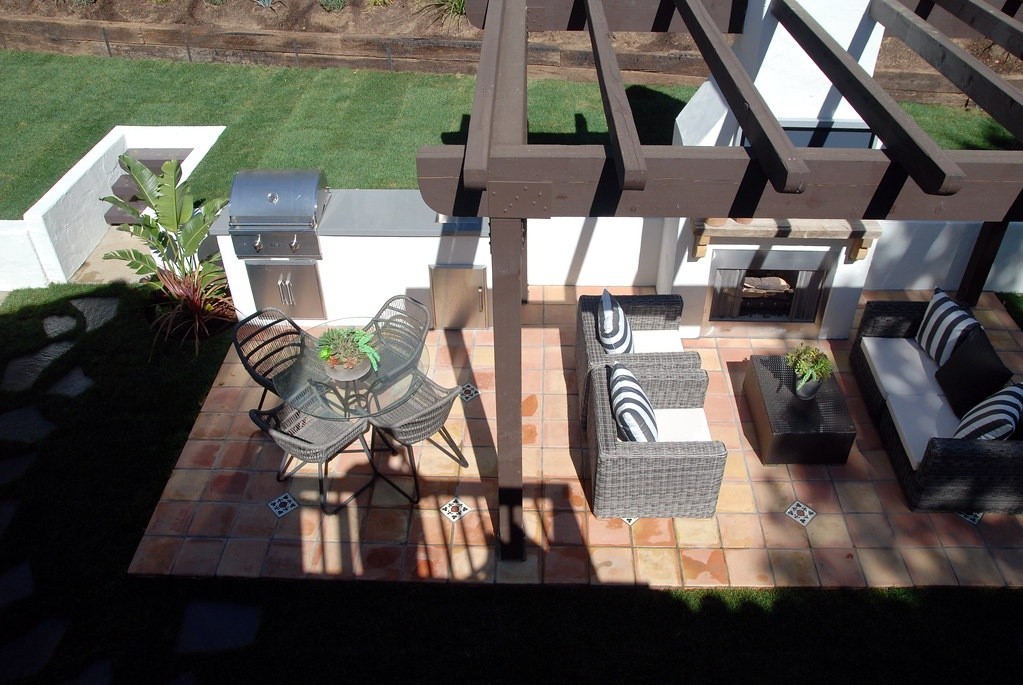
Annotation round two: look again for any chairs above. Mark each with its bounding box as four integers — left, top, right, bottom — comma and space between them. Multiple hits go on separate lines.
248, 378, 379, 516
346, 294, 432, 412
354, 366, 468, 505
233, 307, 338, 415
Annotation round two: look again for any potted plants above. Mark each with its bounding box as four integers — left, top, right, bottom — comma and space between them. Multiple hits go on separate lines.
785, 339, 833, 401
315, 326, 382, 381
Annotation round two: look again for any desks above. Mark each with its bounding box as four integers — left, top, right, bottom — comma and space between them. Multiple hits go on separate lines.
272, 315, 431, 461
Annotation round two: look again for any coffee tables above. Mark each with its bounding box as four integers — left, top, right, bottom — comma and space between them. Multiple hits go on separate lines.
742, 354, 858, 467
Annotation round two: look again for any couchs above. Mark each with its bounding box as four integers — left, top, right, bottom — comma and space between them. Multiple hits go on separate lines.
848, 300, 1023, 515
586, 366, 728, 519
574, 292, 702, 428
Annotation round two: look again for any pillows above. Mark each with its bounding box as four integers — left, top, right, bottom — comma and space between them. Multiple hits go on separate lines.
610, 360, 658, 444
913, 286, 981, 367
597, 287, 636, 356
932, 327, 1015, 422
953, 380, 1023, 439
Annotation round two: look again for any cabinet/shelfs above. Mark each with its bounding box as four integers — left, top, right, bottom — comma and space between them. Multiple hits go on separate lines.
243, 259, 329, 320
429, 263, 489, 331
